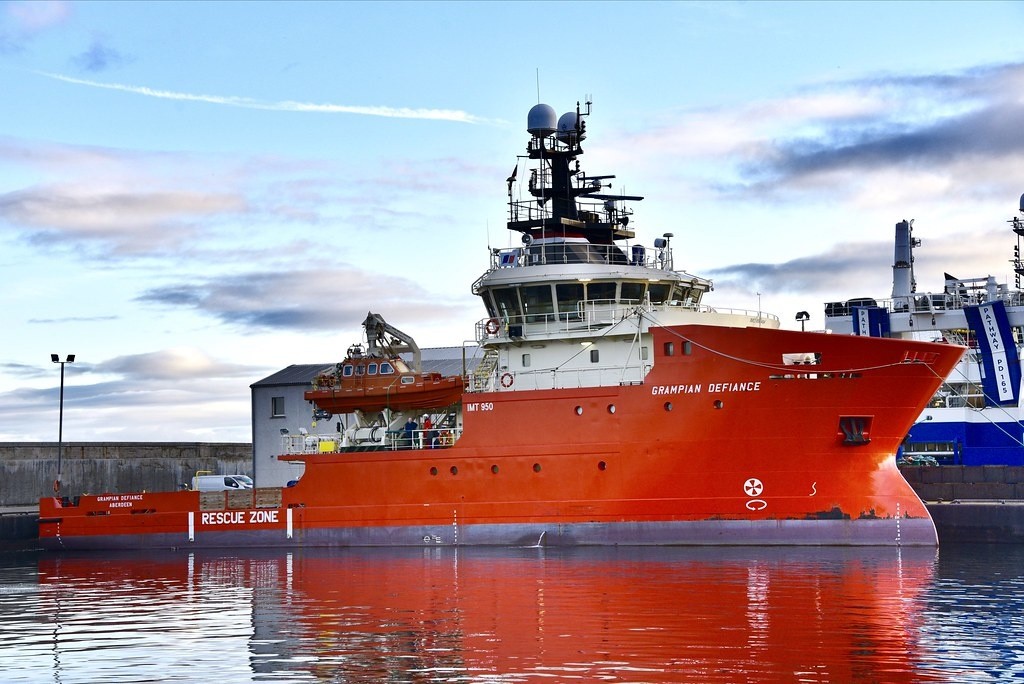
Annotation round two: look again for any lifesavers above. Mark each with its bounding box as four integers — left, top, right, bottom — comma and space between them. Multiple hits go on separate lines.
501, 373, 513, 387
486, 319, 500, 334
439, 431, 451, 445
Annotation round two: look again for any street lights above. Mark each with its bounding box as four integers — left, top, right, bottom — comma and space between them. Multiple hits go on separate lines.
796, 311, 810, 330
51, 354, 76, 498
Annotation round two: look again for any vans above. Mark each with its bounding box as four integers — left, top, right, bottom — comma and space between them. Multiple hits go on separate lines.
192, 475, 252, 494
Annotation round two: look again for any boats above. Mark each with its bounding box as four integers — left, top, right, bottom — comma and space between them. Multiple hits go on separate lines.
824, 193, 1024, 466
33, 69, 969, 546
304, 354, 464, 417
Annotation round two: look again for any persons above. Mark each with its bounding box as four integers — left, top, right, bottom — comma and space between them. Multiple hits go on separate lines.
403, 417, 418, 449
423, 414, 433, 449
